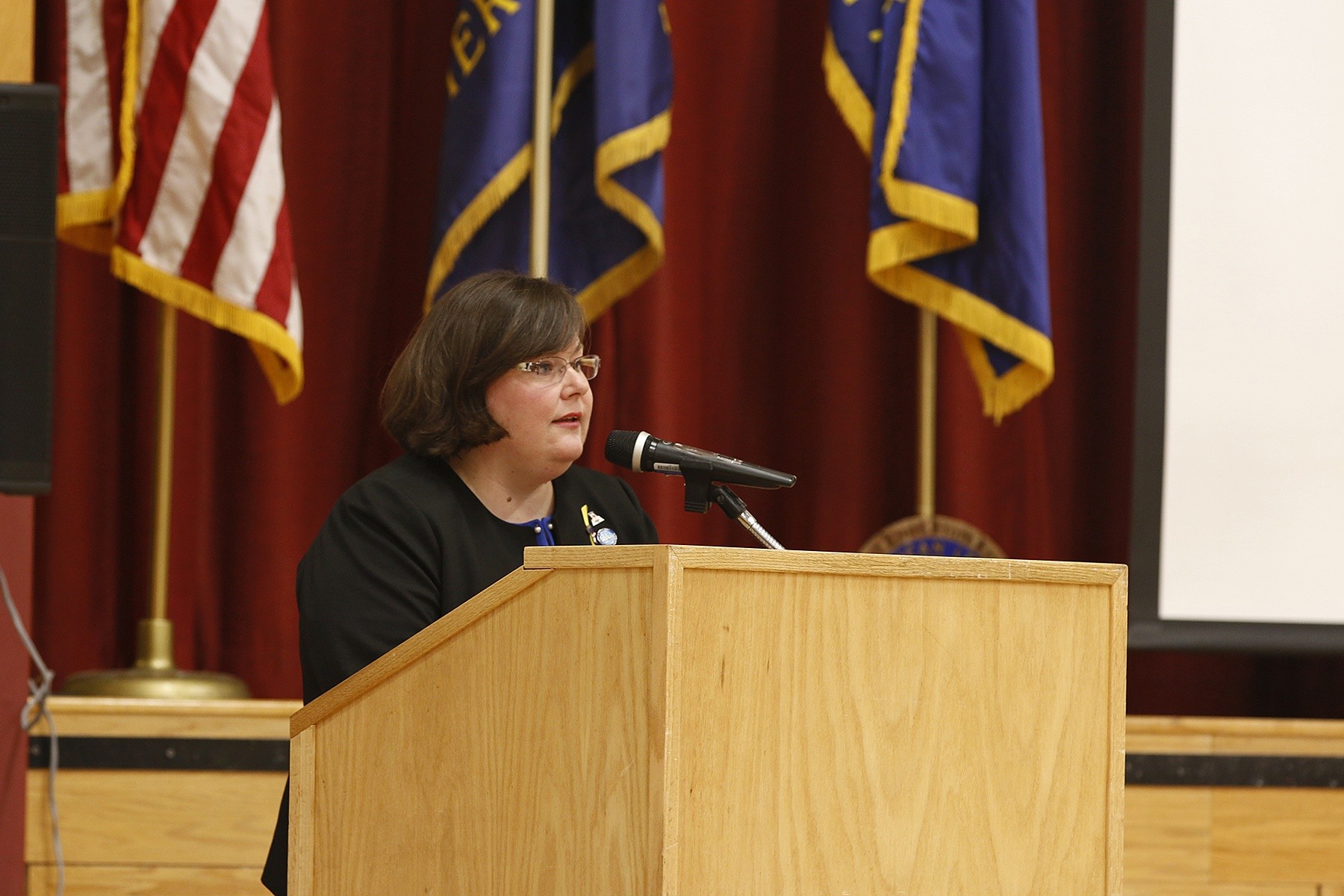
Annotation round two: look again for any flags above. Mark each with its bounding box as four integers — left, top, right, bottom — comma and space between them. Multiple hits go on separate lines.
823, 1, 1054, 426
421, 0, 676, 333
45, 1, 306, 407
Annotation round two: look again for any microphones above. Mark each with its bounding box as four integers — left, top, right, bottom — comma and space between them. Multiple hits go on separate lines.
603, 429, 797, 492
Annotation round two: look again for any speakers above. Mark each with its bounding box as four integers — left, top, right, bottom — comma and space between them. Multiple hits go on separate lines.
0, 80, 62, 496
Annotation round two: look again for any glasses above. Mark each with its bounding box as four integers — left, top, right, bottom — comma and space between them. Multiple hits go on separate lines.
512, 354, 601, 383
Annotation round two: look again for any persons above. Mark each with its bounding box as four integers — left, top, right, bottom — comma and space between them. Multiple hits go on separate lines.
260, 272, 660, 896
